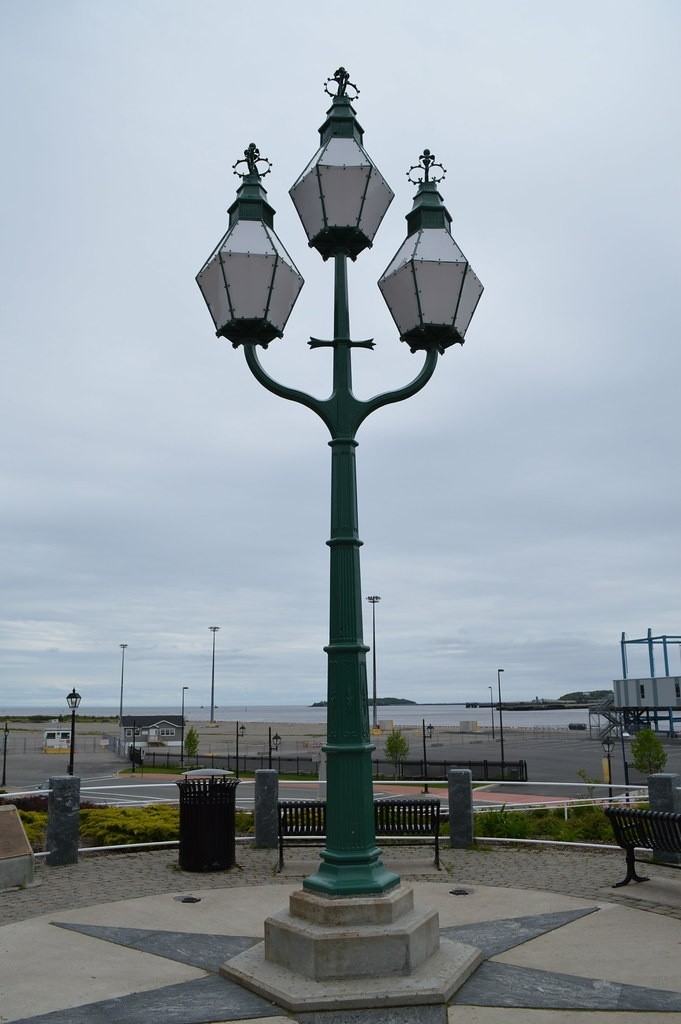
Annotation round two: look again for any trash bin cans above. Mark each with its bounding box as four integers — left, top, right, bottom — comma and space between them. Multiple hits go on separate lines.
173, 768, 241, 872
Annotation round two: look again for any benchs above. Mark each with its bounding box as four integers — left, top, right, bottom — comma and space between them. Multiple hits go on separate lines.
277, 799, 441, 872
604, 807, 681, 889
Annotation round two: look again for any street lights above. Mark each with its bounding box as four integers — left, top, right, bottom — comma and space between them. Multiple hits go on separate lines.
0, 724, 8, 783
208, 626, 220, 723
119, 643, 128, 726
489, 687, 495, 739
268, 727, 281, 768
66, 687, 82, 776
193, 66, 487, 1014
182, 687, 188, 762
498, 669, 504, 779
423, 719, 435, 794
367, 595, 380, 727
602, 735, 615, 803
236, 722, 246, 778
